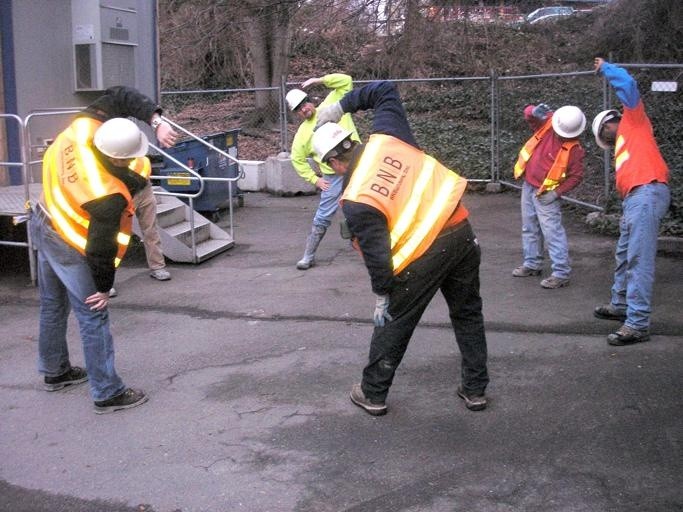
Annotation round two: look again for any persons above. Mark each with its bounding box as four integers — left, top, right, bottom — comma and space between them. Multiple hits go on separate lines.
312, 83, 494, 419
282, 72, 362, 270
592, 57, 671, 346
36, 86, 179, 413
512, 103, 587, 291
110, 157, 175, 298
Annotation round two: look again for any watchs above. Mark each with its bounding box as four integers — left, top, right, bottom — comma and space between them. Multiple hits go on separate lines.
149, 117, 164, 132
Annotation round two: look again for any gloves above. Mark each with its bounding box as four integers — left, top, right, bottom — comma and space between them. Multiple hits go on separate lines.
372, 295, 393, 330
539, 190, 559, 206
311, 103, 345, 129
531, 103, 553, 120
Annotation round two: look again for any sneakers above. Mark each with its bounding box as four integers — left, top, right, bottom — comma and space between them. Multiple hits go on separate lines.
592, 304, 627, 324
455, 384, 487, 411
513, 265, 542, 278
91, 385, 149, 416
108, 287, 118, 298
349, 384, 387, 417
148, 270, 171, 281
540, 274, 573, 289
607, 325, 652, 345
42, 364, 91, 392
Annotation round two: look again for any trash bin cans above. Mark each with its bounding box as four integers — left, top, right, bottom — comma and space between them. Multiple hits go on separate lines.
156, 129, 245, 223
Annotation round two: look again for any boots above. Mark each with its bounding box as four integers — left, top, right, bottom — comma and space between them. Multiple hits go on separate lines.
295, 225, 328, 270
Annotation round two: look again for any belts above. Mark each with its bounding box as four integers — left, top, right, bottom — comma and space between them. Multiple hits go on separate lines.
431, 219, 471, 237
35, 203, 54, 231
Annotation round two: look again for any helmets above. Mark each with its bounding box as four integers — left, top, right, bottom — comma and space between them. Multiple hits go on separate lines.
591, 109, 623, 151
310, 122, 355, 163
552, 105, 588, 139
93, 116, 149, 158
285, 89, 310, 112
